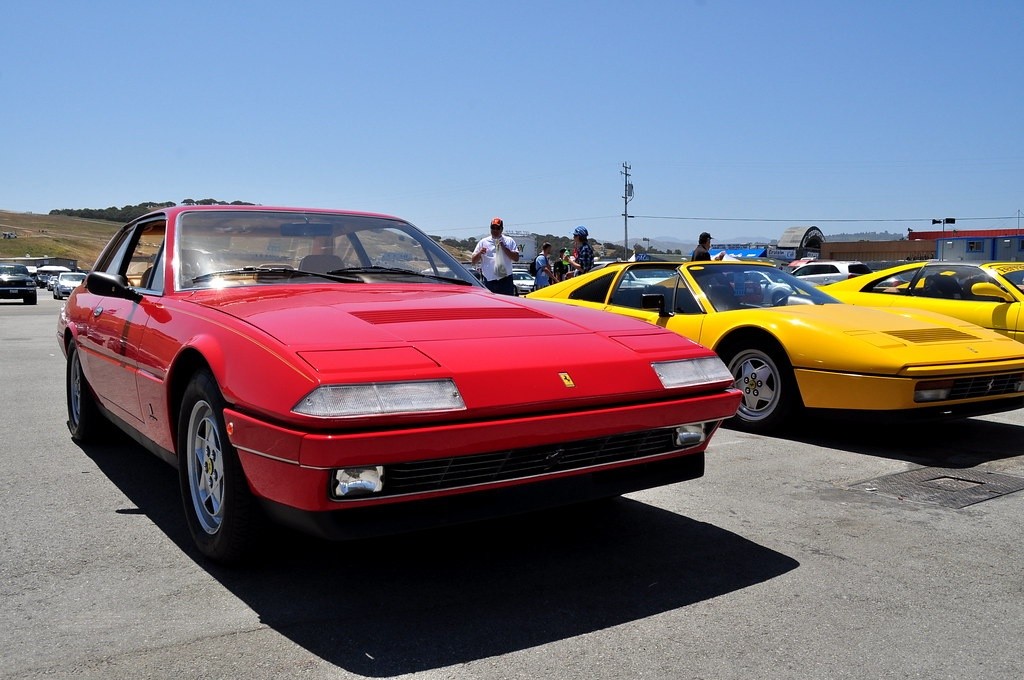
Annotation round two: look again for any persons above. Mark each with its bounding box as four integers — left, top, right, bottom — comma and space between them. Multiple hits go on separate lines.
472, 218, 520, 295
691, 232, 725, 260
925, 275, 943, 297
534, 227, 593, 290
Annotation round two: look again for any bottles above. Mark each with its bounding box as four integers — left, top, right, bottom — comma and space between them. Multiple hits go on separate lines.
562, 247, 571, 265
715, 250, 725, 261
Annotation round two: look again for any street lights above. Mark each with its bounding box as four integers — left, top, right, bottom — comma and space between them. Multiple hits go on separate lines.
932, 218, 954, 262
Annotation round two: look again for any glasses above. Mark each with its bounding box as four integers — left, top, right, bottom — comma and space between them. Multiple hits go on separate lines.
491, 226, 501, 230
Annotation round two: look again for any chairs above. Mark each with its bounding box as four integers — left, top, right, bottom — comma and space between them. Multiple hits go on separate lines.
707, 285, 743, 311
963, 280, 989, 301
140, 263, 193, 289
298, 255, 345, 274
924, 275, 964, 300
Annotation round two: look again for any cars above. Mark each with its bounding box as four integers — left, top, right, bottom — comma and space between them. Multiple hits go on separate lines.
512, 269, 536, 295
866, 263, 932, 289
789, 263, 874, 286
588, 262, 680, 290
31, 272, 88, 300
422, 266, 484, 283
725, 269, 822, 307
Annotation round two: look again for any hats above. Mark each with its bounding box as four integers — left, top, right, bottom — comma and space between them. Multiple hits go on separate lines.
560, 247, 570, 253
700, 232, 714, 240
573, 226, 588, 238
492, 217, 502, 226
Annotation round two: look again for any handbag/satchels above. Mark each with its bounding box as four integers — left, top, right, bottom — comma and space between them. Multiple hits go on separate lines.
492, 243, 508, 277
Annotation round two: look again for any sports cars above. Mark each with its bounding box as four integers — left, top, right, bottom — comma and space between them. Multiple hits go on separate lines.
517, 259, 1024, 429
55, 201, 743, 553
802, 262, 1024, 344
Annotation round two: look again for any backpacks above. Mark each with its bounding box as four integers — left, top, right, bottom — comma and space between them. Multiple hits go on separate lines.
530, 254, 547, 277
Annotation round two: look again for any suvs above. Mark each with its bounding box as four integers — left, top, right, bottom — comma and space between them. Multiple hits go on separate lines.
0, 264, 38, 305
